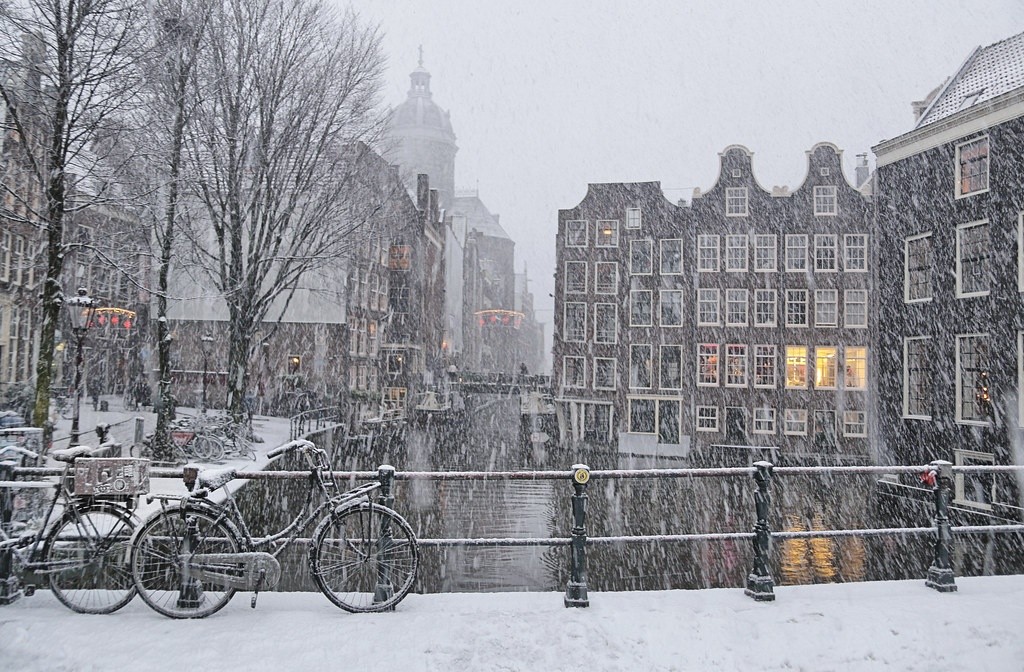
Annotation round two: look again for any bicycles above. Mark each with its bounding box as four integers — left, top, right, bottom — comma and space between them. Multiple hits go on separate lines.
122, 390, 138, 412
130, 411, 257, 466
0, 381, 75, 426
0, 444, 151, 615
123, 439, 420, 619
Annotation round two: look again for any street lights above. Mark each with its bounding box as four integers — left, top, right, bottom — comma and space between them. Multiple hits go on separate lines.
200, 334, 214, 412
66, 287, 101, 450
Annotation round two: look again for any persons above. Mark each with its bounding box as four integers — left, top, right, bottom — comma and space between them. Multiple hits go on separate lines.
517, 360, 530, 375
87, 375, 158, 417
